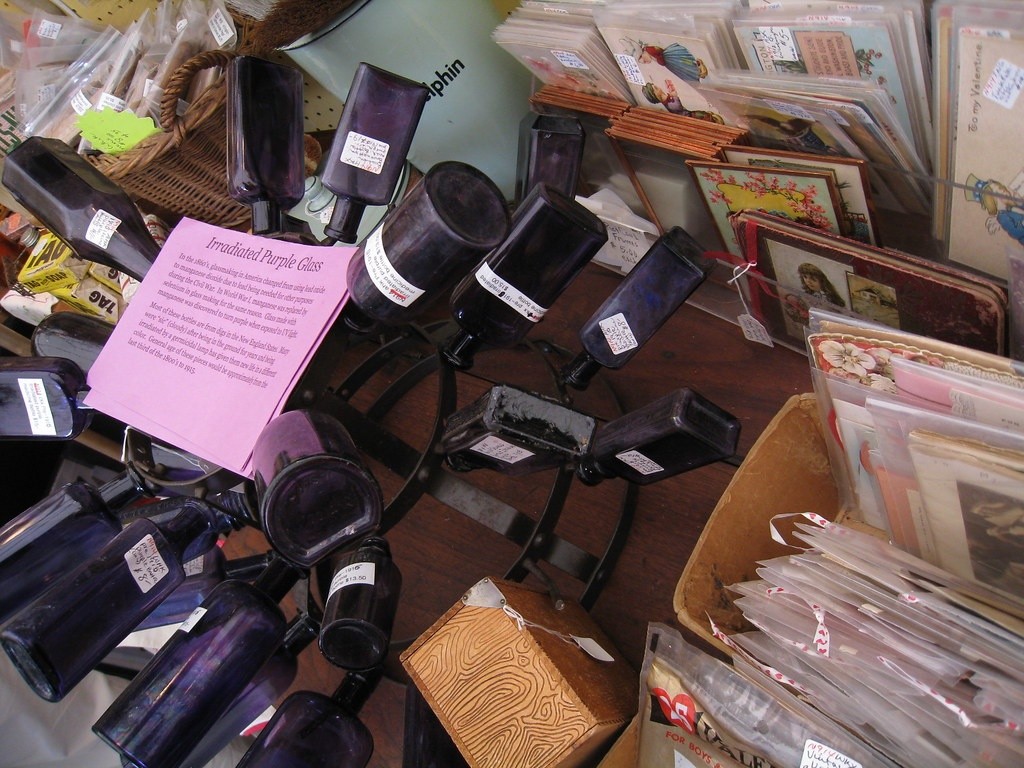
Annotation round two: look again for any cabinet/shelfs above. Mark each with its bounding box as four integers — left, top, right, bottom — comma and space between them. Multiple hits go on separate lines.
127, 235, 643, 679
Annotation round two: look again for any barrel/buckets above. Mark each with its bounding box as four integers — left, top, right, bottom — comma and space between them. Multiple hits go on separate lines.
275, 1, 539, 204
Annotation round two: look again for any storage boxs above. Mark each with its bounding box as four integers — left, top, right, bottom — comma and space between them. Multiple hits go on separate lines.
19, 232, 127, 320
399, 577, 641, 768
673, 392, 838, 661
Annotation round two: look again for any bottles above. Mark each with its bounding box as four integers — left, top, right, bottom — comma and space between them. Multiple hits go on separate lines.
0, 54, 742, 768
